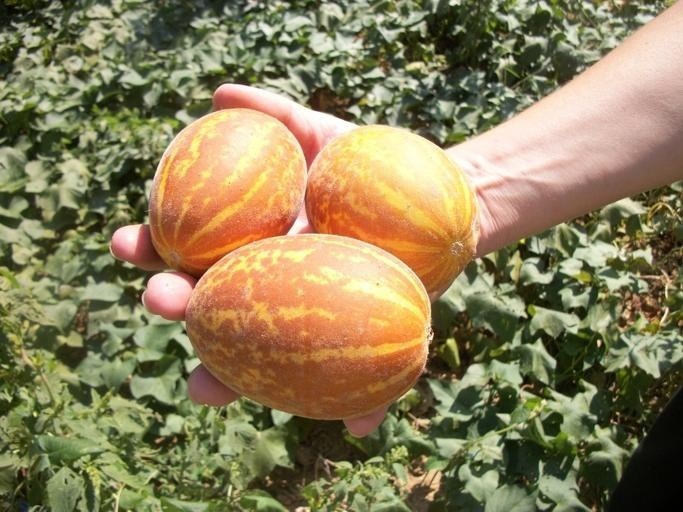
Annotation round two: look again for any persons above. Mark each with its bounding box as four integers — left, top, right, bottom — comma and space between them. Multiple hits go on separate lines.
109, 0, 682, 510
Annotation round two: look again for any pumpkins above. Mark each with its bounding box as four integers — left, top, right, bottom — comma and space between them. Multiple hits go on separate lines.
183, 233, 437, 422
148, 108, 306, 282
306, 123, 476, 306
